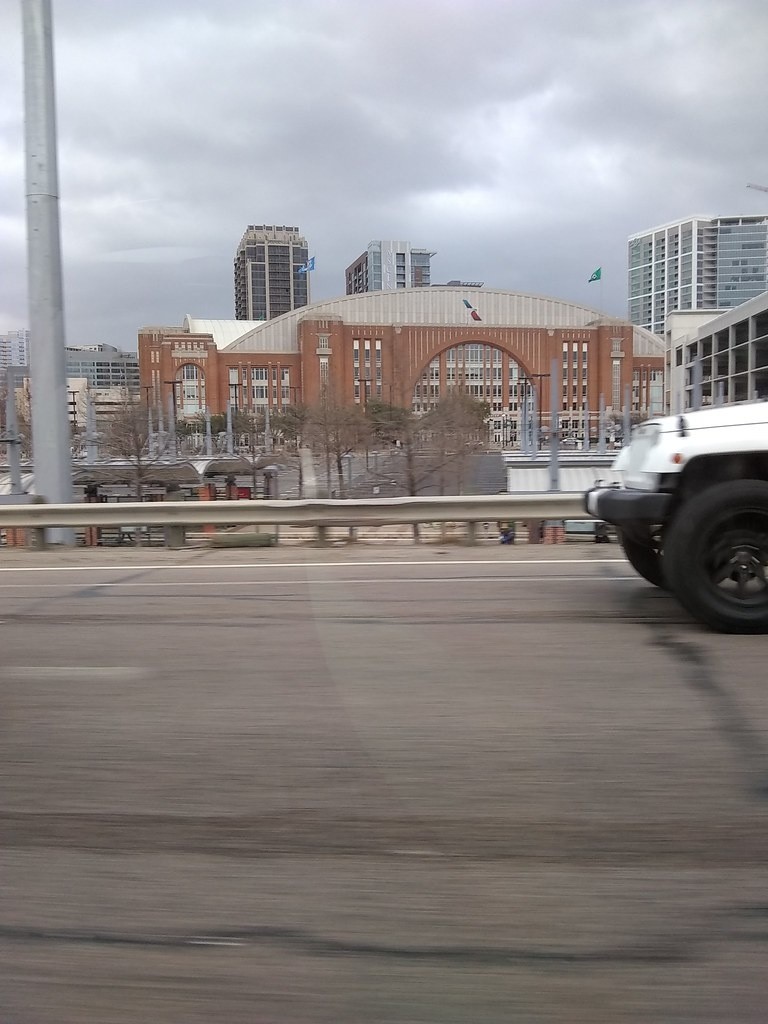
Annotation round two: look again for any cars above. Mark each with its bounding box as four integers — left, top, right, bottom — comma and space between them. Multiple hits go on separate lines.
585, 396, 768, 635
614, 438, 624, 450
561, 438, 577, 445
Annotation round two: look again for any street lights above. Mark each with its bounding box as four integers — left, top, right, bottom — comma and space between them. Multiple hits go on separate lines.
143, 385, 154, 435
228, 384, 243, 431
532, 373, 550, 450
164, 380, 181, 457
516, 377, 533, 447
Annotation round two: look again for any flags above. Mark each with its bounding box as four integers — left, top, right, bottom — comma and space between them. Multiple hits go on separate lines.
588, 267, 601, 282
297, 257, 314, 272
